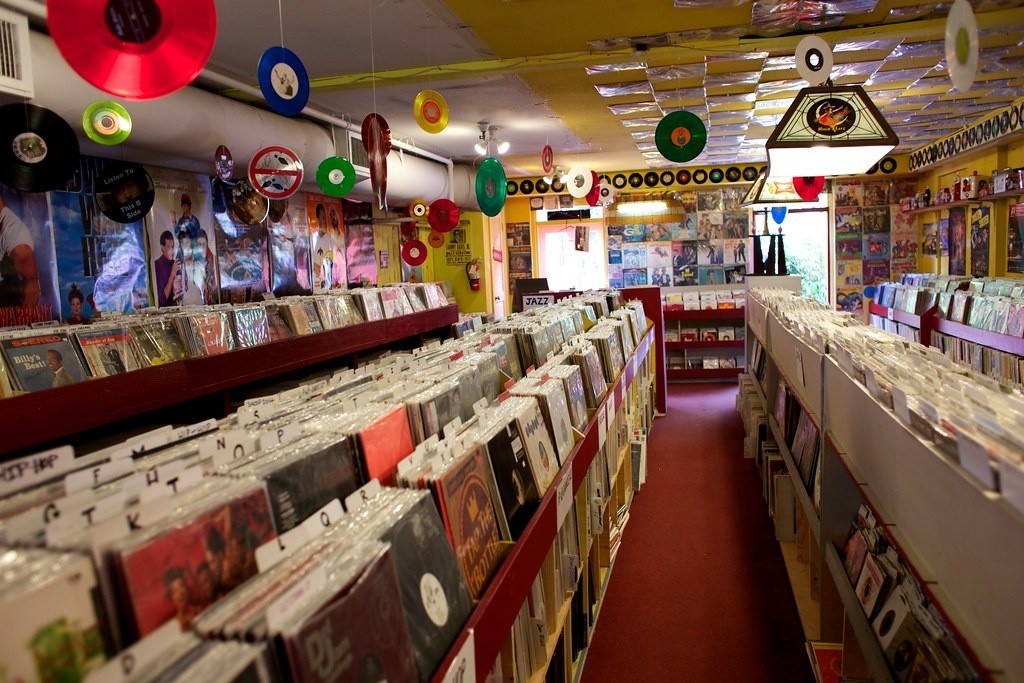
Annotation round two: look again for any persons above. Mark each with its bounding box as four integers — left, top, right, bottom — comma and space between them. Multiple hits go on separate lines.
311, 203, 346, 287
64, 283, 89, 324
48, 349, 75, 387
0, 199, 41, 316
607, 194, 746, 287
155, 195, 215, 307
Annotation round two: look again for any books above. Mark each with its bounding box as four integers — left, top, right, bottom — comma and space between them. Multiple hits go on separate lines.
0, 274, 1024, 683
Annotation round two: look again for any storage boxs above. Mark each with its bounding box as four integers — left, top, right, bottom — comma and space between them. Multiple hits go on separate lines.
960, 175, 989, 200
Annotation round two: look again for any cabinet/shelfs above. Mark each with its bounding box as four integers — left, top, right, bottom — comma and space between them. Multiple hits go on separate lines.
1, 285, 666, 683
664, 309, 744, 383
744, 277, 1024, 683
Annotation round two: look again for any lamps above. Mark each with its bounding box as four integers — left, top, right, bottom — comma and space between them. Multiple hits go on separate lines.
475, 124, 510, 156
543, 165, 568, 185
